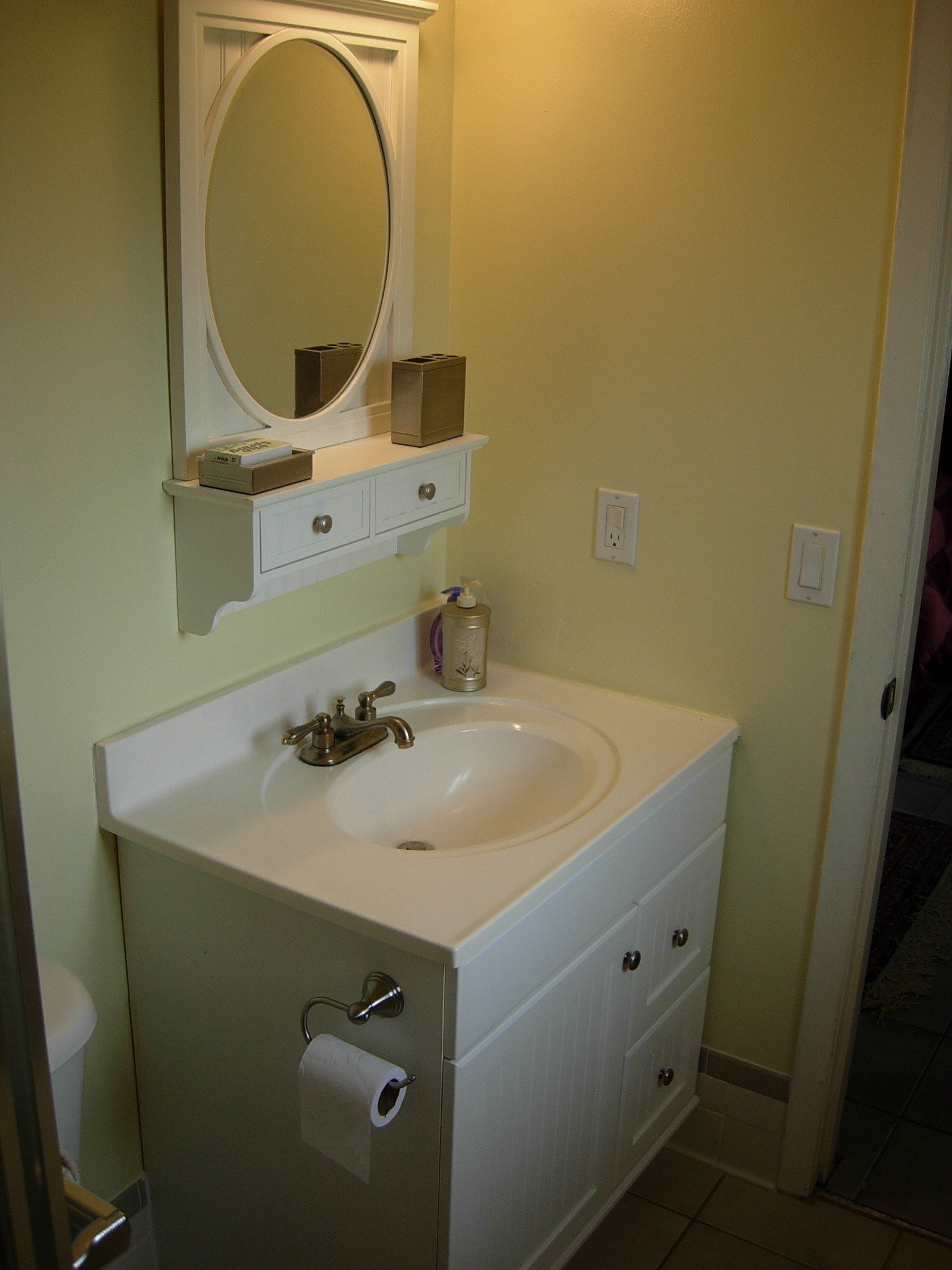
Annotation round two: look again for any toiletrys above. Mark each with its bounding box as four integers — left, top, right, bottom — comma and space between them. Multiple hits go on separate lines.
427, 575, 491, 694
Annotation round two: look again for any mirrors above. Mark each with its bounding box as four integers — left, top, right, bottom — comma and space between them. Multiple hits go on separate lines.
206, 40, 389, 419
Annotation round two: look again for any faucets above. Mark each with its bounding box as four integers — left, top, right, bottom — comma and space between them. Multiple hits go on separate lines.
281, 679, 418, 767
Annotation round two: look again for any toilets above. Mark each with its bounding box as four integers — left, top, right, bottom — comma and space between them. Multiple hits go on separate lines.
31, 956, 98, 1191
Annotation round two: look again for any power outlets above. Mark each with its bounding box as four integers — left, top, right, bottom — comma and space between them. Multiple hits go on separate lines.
592, 487, 640, 568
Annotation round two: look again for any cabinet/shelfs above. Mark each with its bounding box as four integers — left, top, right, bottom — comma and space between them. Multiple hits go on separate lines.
440, 749, 737, 1270
257, 449, 474, 580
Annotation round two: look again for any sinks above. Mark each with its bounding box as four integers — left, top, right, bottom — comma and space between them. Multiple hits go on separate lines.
254, 691, 623, 862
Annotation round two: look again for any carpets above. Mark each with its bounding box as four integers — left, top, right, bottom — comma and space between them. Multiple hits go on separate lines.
861, 811, 952, 1026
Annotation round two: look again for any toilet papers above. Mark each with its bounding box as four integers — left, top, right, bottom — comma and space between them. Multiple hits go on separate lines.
296, 1030, 409, 1184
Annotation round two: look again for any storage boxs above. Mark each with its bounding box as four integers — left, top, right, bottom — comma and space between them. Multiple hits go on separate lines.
194, 438, 317, 496
